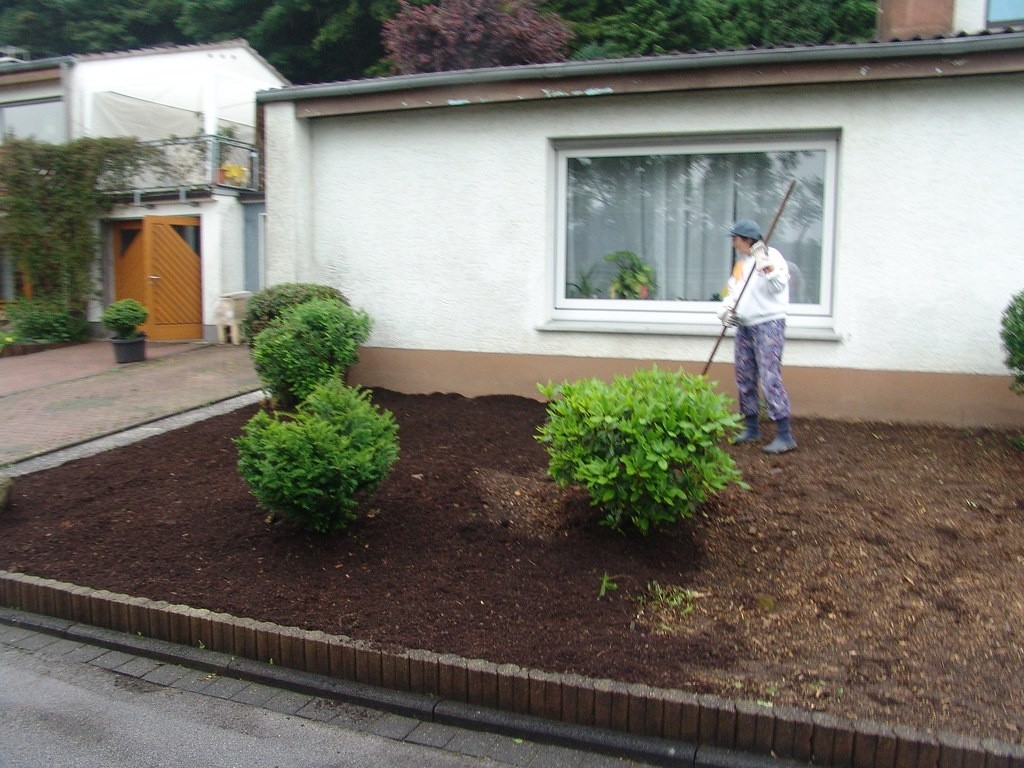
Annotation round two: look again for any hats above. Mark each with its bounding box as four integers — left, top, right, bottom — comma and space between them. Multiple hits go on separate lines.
729, 219, 761, 240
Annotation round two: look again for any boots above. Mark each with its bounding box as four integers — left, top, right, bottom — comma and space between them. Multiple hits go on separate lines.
762, 417, 797, 453
736, 415, 761, 442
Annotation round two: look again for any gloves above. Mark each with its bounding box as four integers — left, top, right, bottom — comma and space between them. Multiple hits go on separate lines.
750, 240, 773, 270
717, 305, 743, 328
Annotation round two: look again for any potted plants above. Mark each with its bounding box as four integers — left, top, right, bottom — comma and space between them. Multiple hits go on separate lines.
102, 296, 148, 363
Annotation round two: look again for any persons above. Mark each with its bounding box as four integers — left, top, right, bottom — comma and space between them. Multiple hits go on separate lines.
716, 218, 798, 454
